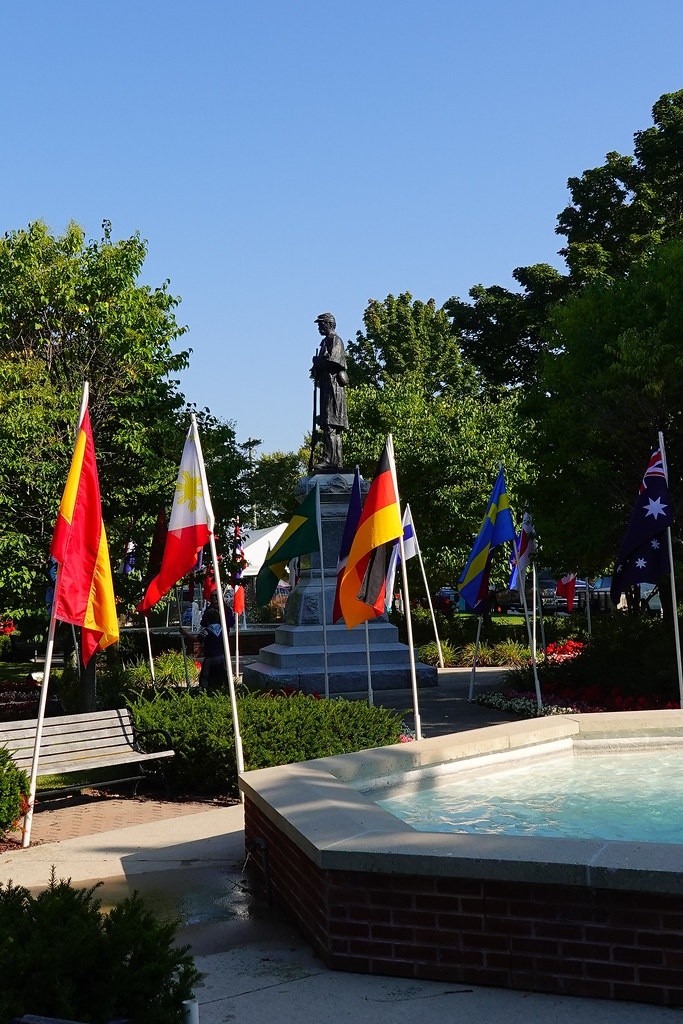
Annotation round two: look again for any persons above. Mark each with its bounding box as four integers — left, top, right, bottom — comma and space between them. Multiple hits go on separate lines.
200, 587, 233, 658
179, 608, 225, 693
308, 312, 349, 469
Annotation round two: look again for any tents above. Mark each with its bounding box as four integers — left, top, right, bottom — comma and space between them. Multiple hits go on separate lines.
220, 522, 298, 629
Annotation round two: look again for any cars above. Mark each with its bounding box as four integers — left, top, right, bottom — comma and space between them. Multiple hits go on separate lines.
421, 586, 461, 611
556, 579, 595, 610
488, 587, 523, 615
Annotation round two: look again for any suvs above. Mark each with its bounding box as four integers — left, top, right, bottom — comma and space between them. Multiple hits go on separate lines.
593, 576, 662, 618
512, 578, 559, 614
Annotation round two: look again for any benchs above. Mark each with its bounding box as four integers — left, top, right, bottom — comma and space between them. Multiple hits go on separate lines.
0, 709, 175, 800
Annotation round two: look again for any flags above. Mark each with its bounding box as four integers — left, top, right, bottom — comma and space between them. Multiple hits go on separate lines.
253, 480, 322, 606
330, 432, 423, 632
613, 444, 674, 606
139, 416, 216, 617
457, 465, 575, 616
48, 383, 119, 669
